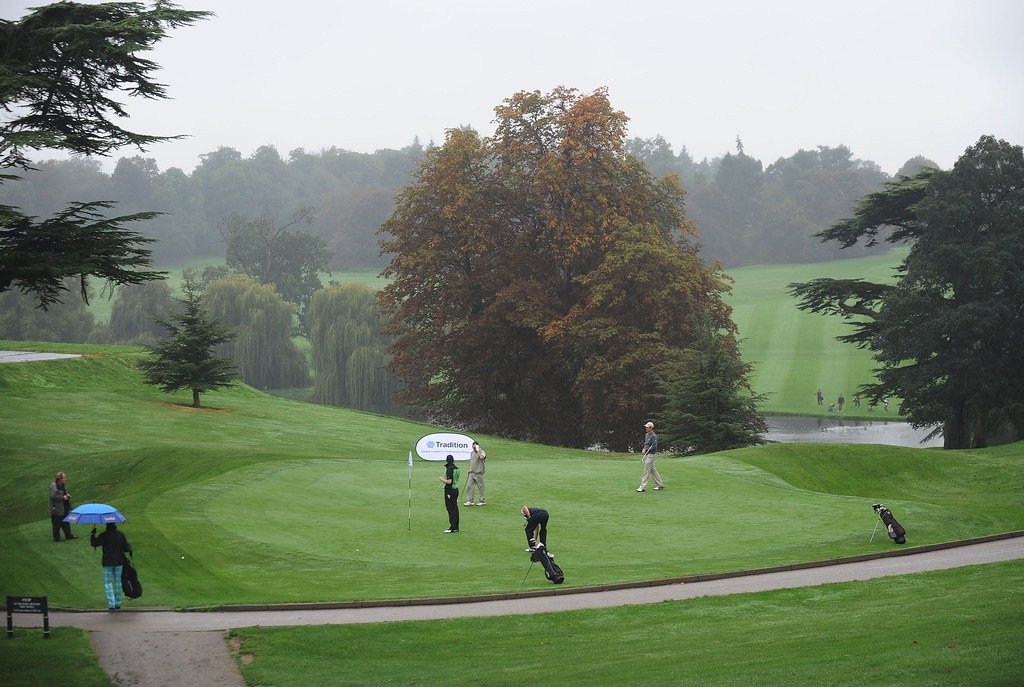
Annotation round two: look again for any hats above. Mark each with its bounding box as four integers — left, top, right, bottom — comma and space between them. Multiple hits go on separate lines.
644, 422, 654, 428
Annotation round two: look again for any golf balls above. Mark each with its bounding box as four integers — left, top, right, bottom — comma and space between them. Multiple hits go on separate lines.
182, 557, 184, 559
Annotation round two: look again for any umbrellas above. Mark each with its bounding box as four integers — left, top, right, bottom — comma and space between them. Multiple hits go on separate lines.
63, 501, 126, 550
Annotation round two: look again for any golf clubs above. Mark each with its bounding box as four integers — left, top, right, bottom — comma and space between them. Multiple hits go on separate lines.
639, 440, 658, 464
523, 523, 539, 545
459, 471, 472, 498
429, 481, 446, 501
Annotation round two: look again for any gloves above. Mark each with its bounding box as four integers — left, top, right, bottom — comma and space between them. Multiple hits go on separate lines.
91, 528, 97, 534
476, 445, 480, 452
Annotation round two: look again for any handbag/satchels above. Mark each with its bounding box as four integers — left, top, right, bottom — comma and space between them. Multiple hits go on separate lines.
122, 549, 143, 598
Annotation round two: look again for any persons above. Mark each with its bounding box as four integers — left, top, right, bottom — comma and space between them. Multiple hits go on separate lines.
463, 442, 486, 505
636, 422, 664, 492
90, 522, 131, 609
817, 389, 824, 405
49, 471, 78, 542
521, 506, 549, 551
883, 396, 888, 413
838, 394, 844, 412
853, 392, 861, 408
440, 454, 460, 533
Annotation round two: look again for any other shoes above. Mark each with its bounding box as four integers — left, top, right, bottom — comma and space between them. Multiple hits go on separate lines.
525, 548, 535, 551
444, 528, 459, 532
636, 488, 645, 492
54, 538, 65, 542
463, 500, 474, 506
109, 606, 121, 609
653, 486, 665, 490
66, 536, 78, 539
476, 502, 486, 506
